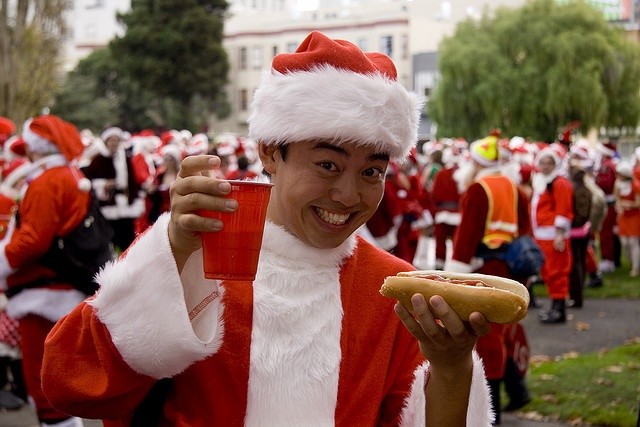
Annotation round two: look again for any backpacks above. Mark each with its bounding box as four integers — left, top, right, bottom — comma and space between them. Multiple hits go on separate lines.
572, 171, 592, 227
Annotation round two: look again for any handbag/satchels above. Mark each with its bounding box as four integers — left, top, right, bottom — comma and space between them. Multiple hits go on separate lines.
505, 236, 543, 283
35, 199, 113, 294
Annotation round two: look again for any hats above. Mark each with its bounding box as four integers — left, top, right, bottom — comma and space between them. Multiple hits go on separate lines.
248, 31, 425, 165
597, 141, 617, 157
101, 126, 125, 143
5, 135, 26, 157
1, 116, 18, 154
469, 129, 500, 167
22, 116, 91, 193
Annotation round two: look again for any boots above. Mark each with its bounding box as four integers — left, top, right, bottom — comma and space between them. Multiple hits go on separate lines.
566, 294, 583, 308
488, 378, 502, 425
587, 272, 602, 287
0, 356, 25, 410
537, 299, 566, 323
503, 357, 530, 413
6, 358, 29, 403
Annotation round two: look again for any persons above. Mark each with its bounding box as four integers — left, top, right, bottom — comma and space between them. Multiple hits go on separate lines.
39, 29, 497, 427
453, 137, 534, 426
449, 139, 640, 323
75, 128, 263, 254
0, 113, 29, 412
367, 135, 446, 271
0, 114, 102, 427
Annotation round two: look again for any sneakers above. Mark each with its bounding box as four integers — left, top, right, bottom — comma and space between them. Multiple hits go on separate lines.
599, 260, 616, 272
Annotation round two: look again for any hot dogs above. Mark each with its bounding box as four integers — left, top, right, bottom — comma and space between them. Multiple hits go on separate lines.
380, 269, 530, 326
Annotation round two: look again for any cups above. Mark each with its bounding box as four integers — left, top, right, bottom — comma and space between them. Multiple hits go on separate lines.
198, 180, 276, 281
